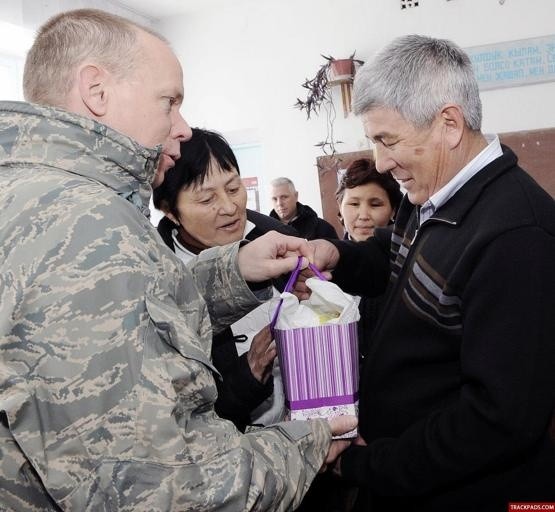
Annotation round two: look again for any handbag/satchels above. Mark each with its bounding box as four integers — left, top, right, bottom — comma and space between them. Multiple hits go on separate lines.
270, 317, 361, 442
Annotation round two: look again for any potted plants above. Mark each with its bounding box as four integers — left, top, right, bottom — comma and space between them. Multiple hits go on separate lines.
292, 48, 366, 179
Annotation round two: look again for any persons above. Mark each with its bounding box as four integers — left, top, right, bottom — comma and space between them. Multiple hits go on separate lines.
291, 37, 555, 512
153, 128, 298, 421
269, 178, 338, 239
0, 9, 358, 512
335, 158, 404, 243
